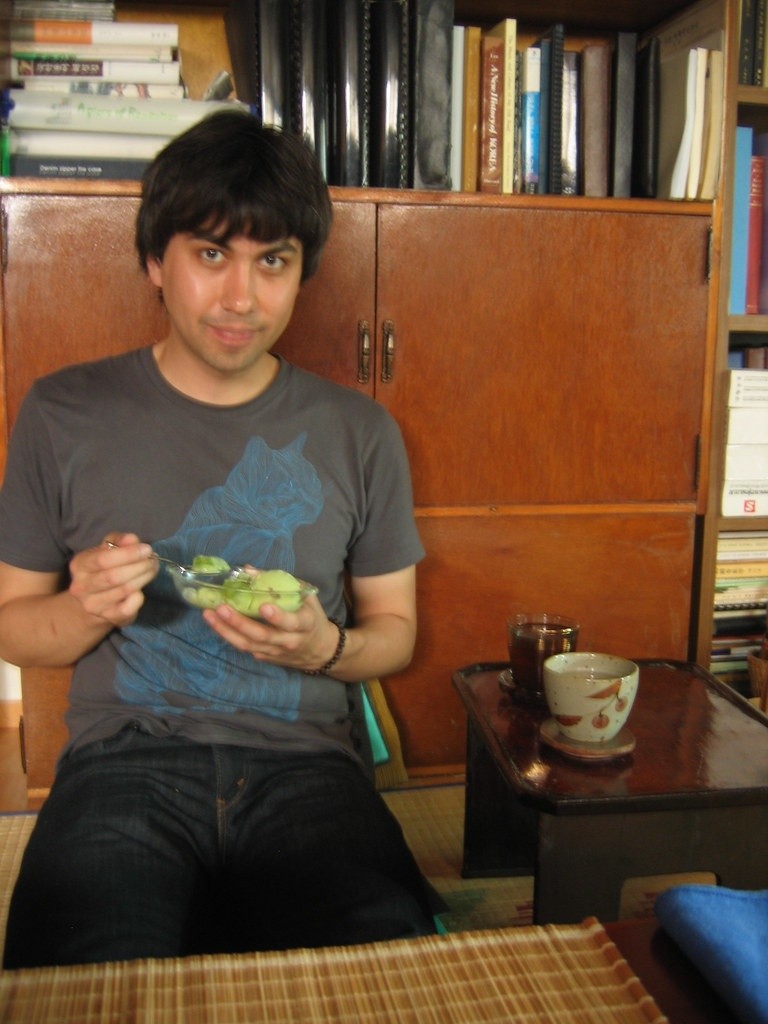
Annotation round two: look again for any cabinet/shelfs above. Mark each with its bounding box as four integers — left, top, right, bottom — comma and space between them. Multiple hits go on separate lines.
0, 0, 768, 806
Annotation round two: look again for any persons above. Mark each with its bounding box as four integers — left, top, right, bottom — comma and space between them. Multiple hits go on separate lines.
0, 109, 452, 971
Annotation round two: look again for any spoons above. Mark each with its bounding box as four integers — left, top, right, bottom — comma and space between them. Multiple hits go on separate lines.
106, 540, 224, 576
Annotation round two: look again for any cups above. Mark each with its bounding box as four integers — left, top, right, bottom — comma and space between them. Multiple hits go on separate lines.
543, 652, 639, 742
748, 649, 768, 697
507, 614, 579, 690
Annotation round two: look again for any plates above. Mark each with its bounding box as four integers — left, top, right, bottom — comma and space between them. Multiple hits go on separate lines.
165, 564, 319, 619
541, 720, 636, 761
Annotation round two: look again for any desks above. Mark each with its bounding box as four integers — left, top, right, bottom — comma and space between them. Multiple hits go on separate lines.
448, 658, 768, 923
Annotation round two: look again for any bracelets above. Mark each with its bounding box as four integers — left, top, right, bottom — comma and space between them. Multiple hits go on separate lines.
305, 616, 346, 675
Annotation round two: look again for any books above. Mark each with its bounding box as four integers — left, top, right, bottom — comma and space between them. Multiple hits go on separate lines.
0, 1, 768, 674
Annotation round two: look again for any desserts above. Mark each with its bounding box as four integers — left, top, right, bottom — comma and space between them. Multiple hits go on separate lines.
184, 554, 301, 616
